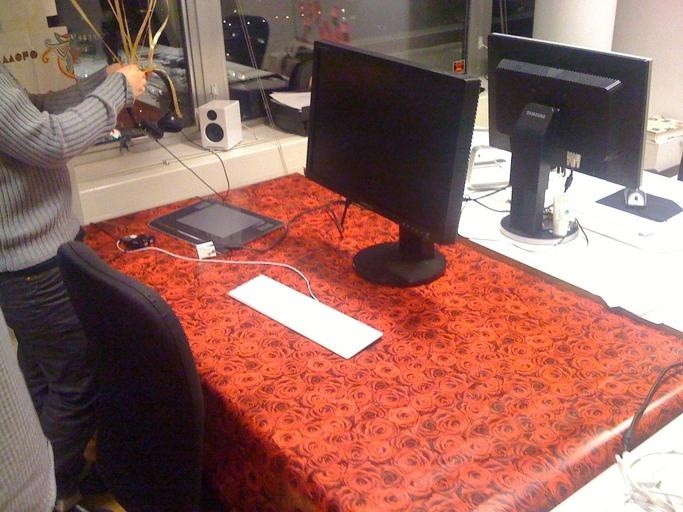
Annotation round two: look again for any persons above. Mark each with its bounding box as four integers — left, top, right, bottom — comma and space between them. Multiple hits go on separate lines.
0, 61, 148, 512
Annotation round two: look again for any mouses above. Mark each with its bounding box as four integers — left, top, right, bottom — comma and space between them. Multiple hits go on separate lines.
624, 188, 647, 207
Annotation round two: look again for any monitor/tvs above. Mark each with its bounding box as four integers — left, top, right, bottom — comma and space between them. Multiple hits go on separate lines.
486, 31, 651, 246
304, 39, 482, 288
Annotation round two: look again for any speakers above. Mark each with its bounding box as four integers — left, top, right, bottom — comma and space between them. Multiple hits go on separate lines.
197, 99, 242, 152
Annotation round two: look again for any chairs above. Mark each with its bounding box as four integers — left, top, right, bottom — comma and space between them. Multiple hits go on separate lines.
57, 241, 205, 512
223, 15, 268, 69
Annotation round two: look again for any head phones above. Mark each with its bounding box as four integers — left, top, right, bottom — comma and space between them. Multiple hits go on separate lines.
126, 67, 185, 139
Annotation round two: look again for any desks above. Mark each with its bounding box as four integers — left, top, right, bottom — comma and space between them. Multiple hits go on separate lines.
115, 43, 291, 131
458, 129, 683, 337
83, 171, 683, 512
